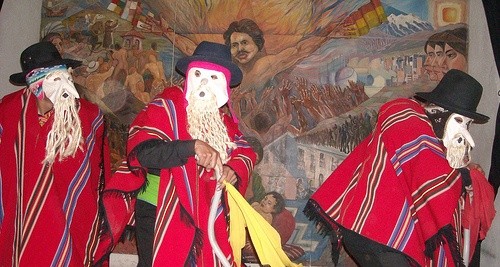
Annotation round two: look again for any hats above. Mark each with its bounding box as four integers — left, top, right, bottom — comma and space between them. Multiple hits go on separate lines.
174, 41, 243, 87
413, 69, 489, 125
10, 41, 82, 86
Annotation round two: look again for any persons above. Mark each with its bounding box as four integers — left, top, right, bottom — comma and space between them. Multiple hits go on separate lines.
0, 41, 112, 267
98, 41, 258, 267
303, 68, 491, 267
